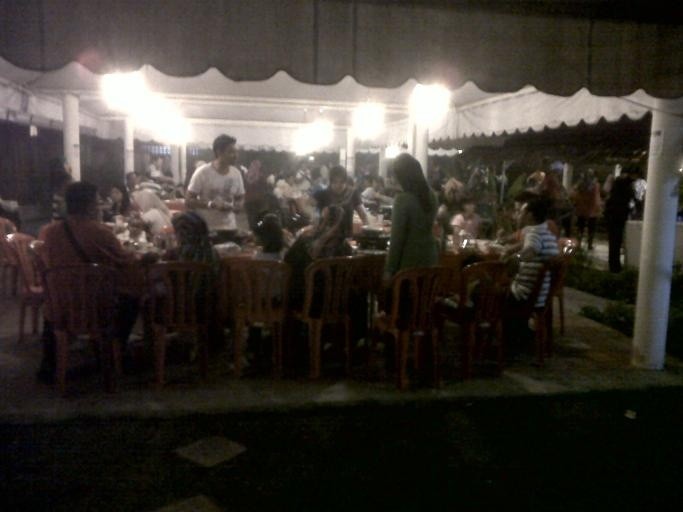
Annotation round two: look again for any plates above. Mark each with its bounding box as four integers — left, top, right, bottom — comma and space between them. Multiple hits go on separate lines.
361, 224, 389, 237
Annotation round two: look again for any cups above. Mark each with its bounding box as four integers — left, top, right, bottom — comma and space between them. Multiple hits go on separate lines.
459, 233, 475, 256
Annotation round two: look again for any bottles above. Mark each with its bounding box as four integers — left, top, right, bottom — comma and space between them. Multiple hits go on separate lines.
111, 215, 177, 263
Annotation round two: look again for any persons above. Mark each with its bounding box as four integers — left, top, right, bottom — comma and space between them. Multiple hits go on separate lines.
33, 132, 648, 393
